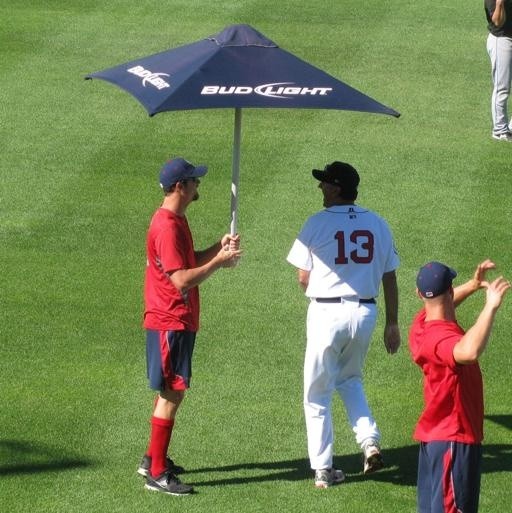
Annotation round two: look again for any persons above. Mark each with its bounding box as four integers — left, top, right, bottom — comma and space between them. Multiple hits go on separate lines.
407, 256, 511, 513
136, 155, 245, 497
483, 0, 512, 141
285, 159, 403, 488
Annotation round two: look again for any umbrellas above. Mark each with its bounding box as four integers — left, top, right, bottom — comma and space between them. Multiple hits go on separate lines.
83, 22, 403, 253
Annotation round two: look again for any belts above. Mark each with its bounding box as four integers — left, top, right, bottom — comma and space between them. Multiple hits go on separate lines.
316, 298, 375, 304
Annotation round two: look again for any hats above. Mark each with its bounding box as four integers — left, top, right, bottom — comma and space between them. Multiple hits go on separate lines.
416, 262, 457, 299
160, 158, 208, 186
312, 161, 360, 186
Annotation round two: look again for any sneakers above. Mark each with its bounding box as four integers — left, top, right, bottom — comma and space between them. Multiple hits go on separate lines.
493, 131, 512, 142
315, 468, 345, 487
137, 454, 184, 476
144, 470, 192, 495
363, 440, 385, 475
